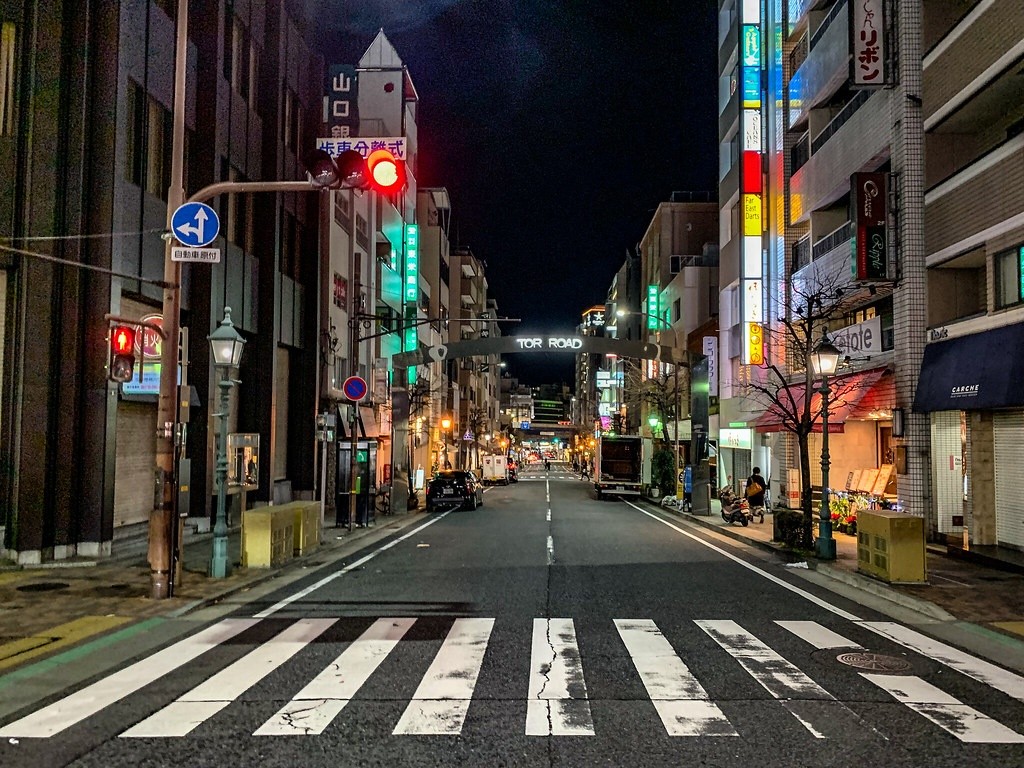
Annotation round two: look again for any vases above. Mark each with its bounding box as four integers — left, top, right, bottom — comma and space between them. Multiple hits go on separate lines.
847, 524, 858, 535
652, 489, 660, 497
832, 519, 838, 530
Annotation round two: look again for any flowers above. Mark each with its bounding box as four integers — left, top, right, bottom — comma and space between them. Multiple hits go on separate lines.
846, 516, 856, 524
831, 514, 841, 520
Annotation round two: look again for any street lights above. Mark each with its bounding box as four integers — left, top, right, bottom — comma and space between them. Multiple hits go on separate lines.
207, 305, 247, 579
647, 410, 660, 451
440, 411, 451, 470
808, 325, 842, 561
617, 309, 678, 495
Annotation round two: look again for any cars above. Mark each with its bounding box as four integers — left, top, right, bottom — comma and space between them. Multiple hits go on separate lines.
426, 469, 483, 513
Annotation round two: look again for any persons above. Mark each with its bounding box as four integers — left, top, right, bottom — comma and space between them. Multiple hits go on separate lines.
744, 467, 766, 524
573, 458, 593, 481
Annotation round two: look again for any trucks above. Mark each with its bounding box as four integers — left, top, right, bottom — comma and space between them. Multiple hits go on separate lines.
482, 454, 518, 486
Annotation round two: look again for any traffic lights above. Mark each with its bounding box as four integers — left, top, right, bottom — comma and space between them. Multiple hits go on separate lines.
302, 146, 407, 191
105, 324, 137, 382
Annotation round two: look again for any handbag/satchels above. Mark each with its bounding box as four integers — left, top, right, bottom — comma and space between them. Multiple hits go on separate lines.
745, 477, 763, 497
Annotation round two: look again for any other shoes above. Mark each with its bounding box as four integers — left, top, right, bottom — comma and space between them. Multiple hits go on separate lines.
581, 478, 582, 481
588, 479, 590, 481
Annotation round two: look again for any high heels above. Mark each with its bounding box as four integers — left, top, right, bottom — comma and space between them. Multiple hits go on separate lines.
760, 519, 764, 524
747, 515, 753, 521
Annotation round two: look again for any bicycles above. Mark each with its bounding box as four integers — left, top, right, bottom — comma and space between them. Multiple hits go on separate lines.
375, 480, 389, 516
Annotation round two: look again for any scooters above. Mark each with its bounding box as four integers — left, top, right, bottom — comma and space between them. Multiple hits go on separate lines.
711, 475, 750, 527
818, 486, 892, 535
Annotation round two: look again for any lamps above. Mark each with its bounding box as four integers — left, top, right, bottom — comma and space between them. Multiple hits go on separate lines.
835, 287, 860, 296
796, 306, 824, 315
869, 284, 877, 297
815, 297, 838, 307
843, 355, 871, 365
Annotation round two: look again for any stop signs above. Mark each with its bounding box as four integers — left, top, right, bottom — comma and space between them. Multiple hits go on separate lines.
343, 375, 369, 402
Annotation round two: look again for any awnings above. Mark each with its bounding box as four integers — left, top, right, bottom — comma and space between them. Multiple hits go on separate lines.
752, 366, 895, 434
911, 322, 1024, 415
